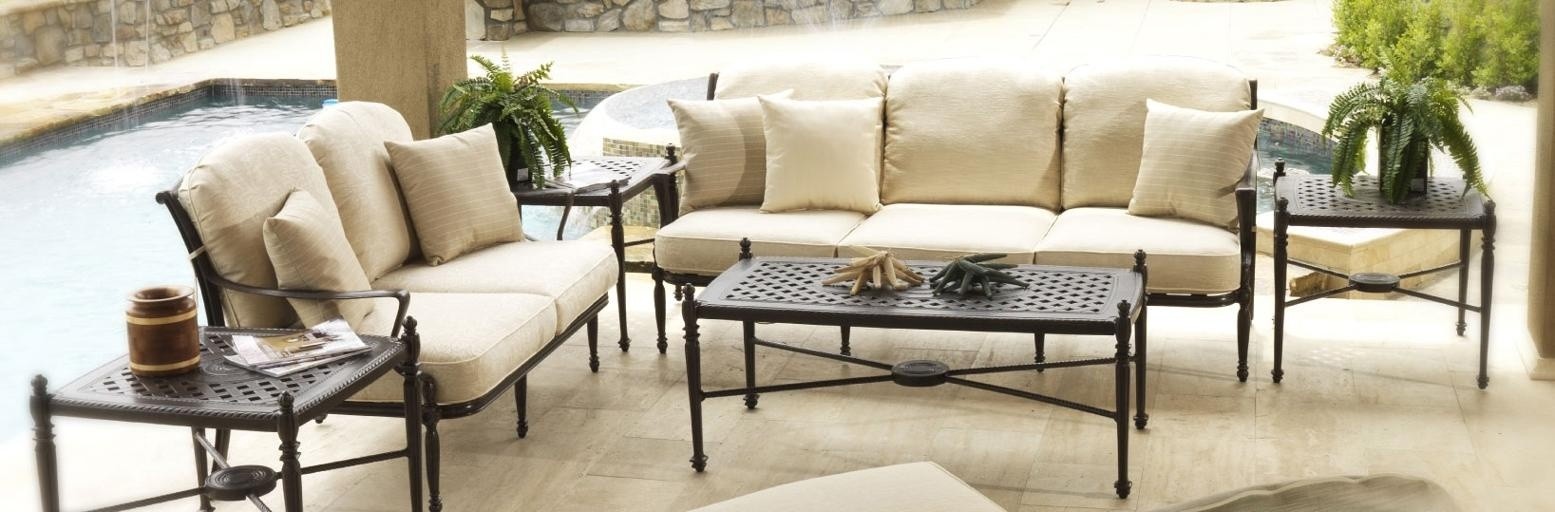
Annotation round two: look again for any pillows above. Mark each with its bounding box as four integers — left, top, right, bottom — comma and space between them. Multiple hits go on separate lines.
263, 192, 377, 334
178, 139, 392, 333
294, 98, 415, 282
1168, 475, 1455, 512
385, 123, 521, 266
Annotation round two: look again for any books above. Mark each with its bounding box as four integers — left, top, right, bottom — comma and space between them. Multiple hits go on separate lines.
223, 318, 372, 378
540, 162, 630, 194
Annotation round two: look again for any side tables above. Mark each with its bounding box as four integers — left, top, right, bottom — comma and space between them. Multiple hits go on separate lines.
30, 316, 421, 511
511, 144, 677, 354
1272, 156, 1496, 388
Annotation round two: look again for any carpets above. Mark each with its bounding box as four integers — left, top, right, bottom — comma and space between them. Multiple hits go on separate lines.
689, 459, 1009, 512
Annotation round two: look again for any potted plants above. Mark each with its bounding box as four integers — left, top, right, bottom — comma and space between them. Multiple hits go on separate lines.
1332, 32, 1495, 204
435, 54, 570, 190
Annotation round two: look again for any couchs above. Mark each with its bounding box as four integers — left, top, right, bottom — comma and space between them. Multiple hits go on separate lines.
156, 102, 619, 512
652, 71, 1256, 383
